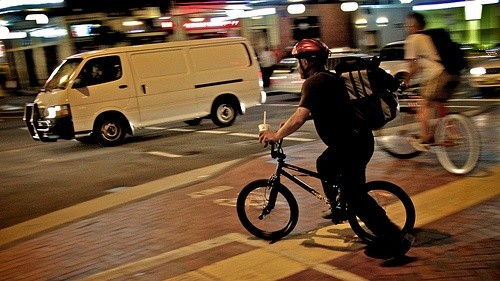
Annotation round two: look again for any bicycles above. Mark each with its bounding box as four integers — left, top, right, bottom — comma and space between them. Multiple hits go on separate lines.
375, 80, 482, 175
236, 122, 416, 243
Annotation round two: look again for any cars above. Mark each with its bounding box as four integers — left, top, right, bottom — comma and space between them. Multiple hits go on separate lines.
267, 40, 500, 98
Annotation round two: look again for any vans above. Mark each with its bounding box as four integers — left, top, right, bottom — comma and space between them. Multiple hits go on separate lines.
23, 36, 267, 147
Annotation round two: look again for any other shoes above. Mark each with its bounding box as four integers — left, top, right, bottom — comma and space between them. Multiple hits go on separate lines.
410, 135, 432, 146
321, 207, 335, 218
387, 234, 415, 258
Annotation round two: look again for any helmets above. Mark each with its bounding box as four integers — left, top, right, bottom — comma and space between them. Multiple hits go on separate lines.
291, 38, 330, 57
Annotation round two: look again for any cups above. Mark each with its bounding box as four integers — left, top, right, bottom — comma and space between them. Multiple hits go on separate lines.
257, 124, 272, 131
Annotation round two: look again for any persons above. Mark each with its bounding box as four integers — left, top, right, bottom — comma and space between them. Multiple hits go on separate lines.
92, 65, 102, 78
260, 48, 277, 92
403, 12, 461, 152
259, 39, 415, 266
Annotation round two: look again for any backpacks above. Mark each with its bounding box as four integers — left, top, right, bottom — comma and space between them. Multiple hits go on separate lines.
319, 56, 400, 130
412, 27, 470, 76
